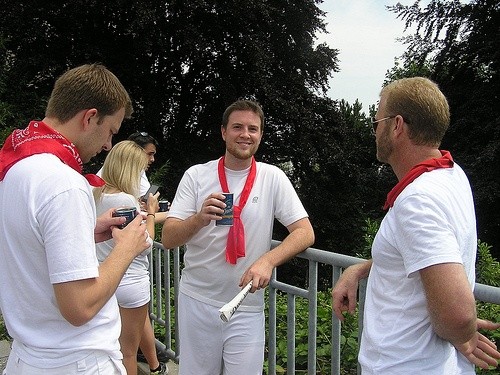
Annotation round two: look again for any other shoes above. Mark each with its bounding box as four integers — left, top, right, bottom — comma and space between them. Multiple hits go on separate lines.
150, 362, 168, 375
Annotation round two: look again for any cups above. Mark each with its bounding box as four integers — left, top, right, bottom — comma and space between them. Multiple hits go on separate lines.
115, 206, 136, 230
216, 193, 233, 227
158, 201, 168, 212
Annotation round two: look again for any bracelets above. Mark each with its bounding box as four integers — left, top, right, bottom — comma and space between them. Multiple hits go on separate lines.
147, 214, 155, 218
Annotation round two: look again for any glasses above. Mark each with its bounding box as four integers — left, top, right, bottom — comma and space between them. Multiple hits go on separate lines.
371, 116, 415, 135
137, 132, 148, 139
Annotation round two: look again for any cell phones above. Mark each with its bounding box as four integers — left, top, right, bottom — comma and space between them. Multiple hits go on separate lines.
143, 185, 159, 200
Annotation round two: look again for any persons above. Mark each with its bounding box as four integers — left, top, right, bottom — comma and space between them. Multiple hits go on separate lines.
331, 77, 500, 375
92, 133, 170, 375
0, 63, 136, 375
162, 97, 315, 375
